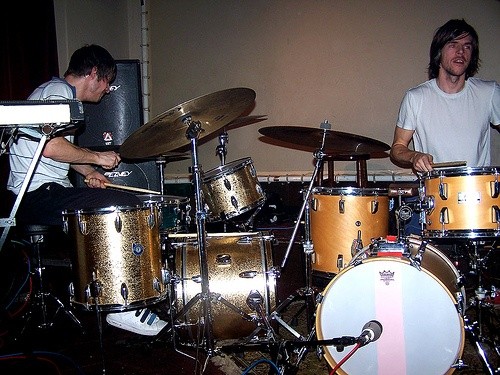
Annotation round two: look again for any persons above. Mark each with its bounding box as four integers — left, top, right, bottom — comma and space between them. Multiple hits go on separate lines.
6, 43, 167, 335
390, 18, 500, 233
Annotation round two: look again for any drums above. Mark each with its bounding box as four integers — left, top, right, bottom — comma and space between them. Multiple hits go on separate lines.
134, 195, 191, 234
314, 234, 466, 375
173, 231, 276, 342
59, 201, 171, 314
301, 186, 390, 275
420, 165, 500, 238
192, 155, 266, 222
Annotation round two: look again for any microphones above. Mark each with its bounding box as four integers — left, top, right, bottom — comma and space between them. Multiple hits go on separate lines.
356, 320, 383, 346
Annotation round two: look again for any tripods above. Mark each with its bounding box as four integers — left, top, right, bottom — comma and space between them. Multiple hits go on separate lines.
0, 124, 500, 375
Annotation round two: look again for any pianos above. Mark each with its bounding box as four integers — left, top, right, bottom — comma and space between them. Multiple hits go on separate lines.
0, 98, 84, 251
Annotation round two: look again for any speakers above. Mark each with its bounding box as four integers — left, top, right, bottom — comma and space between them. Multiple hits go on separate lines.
73, 59, 144, 150
65, 150, 163, 214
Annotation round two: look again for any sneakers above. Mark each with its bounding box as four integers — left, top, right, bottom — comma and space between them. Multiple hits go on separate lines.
106, 308, 168, 336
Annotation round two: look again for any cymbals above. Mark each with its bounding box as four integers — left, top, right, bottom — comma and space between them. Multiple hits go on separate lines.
119, 87, 257, 160
258, 125, 391, 155
160, 151, 191, 157
228, 114, 269, 125
